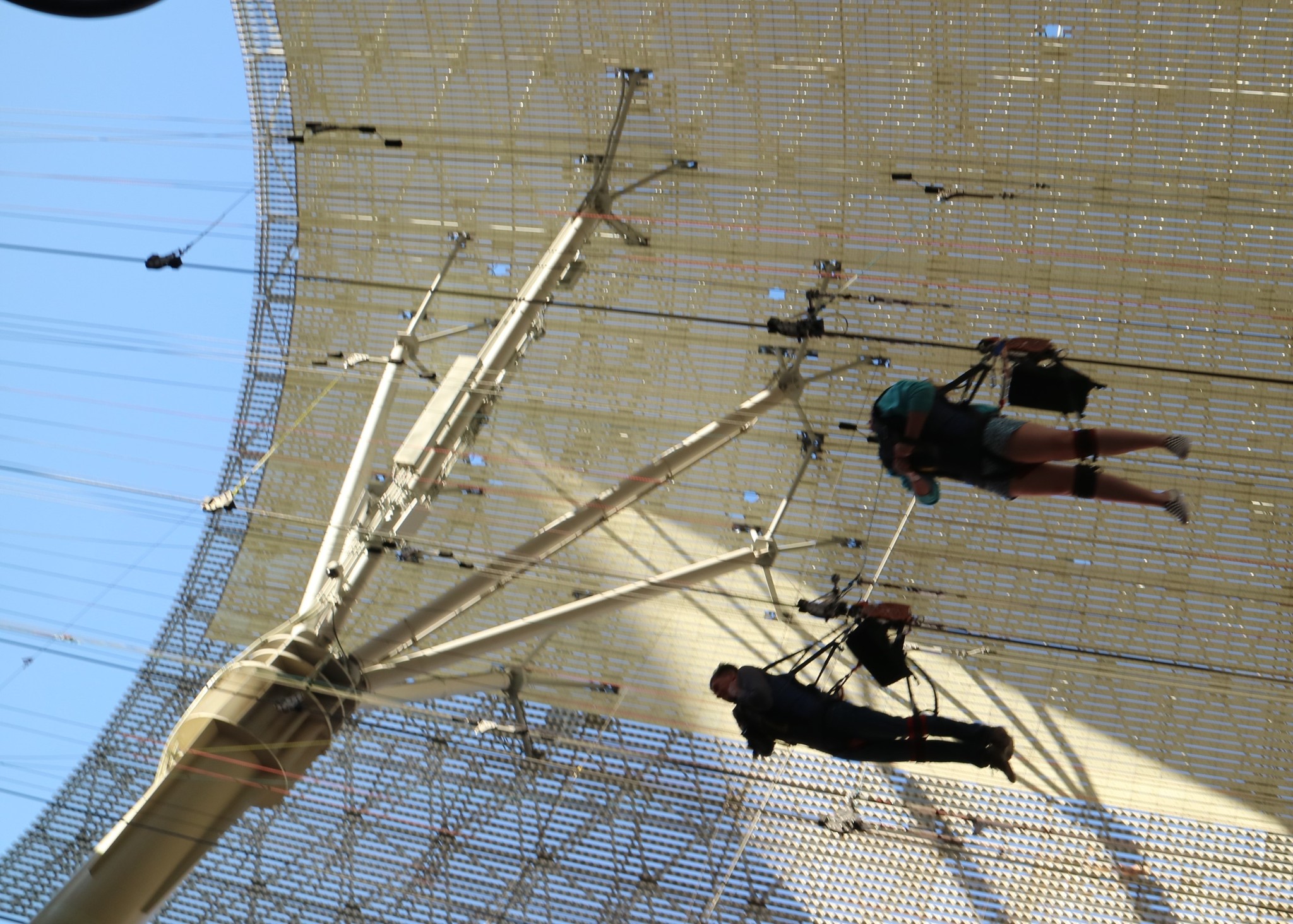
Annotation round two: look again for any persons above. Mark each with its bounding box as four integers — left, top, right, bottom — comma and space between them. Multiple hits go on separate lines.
870, 380, 1191, 526
709, 664, 1016, 784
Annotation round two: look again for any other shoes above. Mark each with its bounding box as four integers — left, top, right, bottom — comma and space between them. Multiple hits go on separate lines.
989, 727, 1015, 782
1164, 434, 1191, 459
1164, 488, 1187, 526
